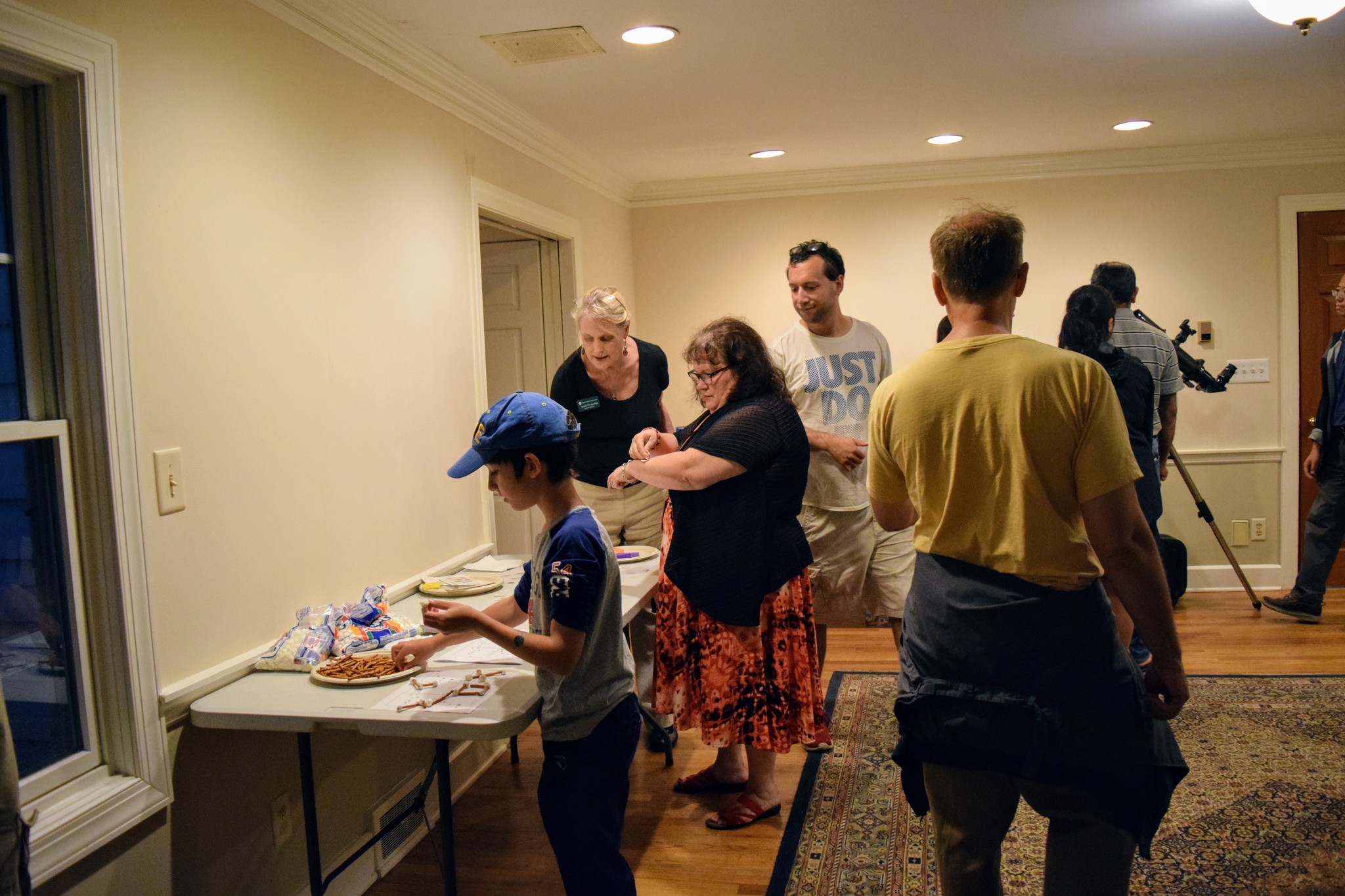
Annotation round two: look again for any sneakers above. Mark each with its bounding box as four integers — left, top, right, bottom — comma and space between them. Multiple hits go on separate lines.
1261, 591, 1325, 623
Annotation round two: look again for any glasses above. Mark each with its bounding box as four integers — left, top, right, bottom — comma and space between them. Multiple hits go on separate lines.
789, 242, 839, 267
1331, 289, 1345, 300
687, 364, 731, 385
574, 291, 627, 317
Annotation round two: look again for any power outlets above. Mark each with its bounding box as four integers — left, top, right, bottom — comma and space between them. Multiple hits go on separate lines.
1252, 519, 1266, 540
1226, 358, 1270, 383
271, 795, 293, 847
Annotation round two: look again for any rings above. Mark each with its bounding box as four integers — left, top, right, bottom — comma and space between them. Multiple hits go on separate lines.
609, 483, 613, 486
629, 449, 632, 454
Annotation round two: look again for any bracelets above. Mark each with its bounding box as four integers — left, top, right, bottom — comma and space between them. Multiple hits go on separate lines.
622, 460, 639, 484
641, 427, 660, 450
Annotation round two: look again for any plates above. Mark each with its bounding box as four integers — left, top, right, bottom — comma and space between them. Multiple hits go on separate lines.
613, 545, 659, 563
419, 575, 505, 597
310, 650, 427, 685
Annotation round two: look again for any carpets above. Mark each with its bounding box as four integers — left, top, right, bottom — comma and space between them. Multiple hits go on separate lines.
768, 672, 1345, 896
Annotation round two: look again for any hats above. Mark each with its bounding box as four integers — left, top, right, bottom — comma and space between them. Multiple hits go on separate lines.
447, 390, 581, 480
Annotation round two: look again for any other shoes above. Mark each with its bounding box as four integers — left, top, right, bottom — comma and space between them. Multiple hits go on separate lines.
802, 712, 834, 751
652, 726, 678, 749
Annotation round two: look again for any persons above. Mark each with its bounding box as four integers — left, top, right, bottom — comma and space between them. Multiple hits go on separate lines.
550, 286, 679, 752
768, 240, 916, 753
608, 317, 815, 832
392, 390, 641, 895
1089, 257, 1184, 670
1261, 275, 1345, 623
1054, 286, 1164, 652
870, 207, 1190, 896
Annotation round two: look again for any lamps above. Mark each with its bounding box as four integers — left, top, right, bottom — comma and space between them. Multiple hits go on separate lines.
1249, 0, 1345, 35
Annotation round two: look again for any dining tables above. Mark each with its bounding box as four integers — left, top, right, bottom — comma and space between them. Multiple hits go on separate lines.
188, 548, 660, 896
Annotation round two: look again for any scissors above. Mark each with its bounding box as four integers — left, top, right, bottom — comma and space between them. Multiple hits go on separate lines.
423, 582, 476, 589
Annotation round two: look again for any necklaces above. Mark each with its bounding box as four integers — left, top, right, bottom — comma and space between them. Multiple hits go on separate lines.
599, 370, 619, 400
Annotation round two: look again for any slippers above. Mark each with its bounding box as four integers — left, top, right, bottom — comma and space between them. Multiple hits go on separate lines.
673, 767, 748, 794
705, 791, 781, 830
